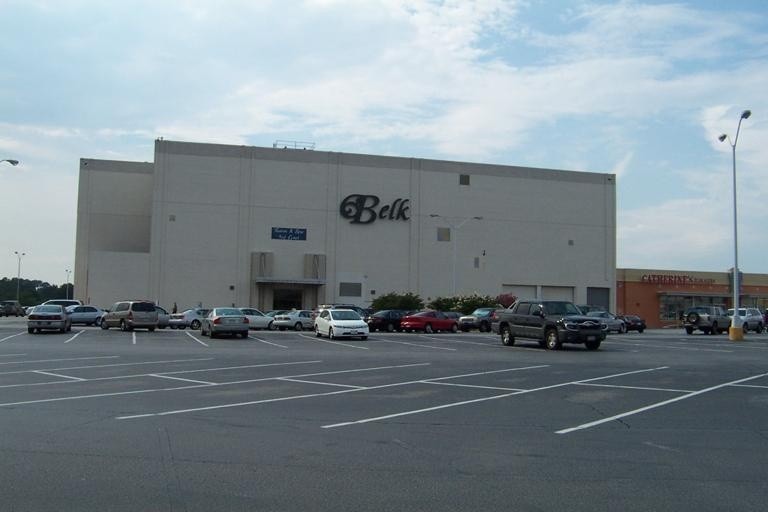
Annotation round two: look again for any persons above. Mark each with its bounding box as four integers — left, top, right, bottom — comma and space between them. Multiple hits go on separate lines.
172, 303, 177, 314
4, 303, 10, 317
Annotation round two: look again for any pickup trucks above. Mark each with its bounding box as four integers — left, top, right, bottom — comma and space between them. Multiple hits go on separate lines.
490, 299, 609, 351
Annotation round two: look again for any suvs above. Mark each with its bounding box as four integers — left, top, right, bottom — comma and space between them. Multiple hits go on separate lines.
683, 306, 768, 335
458, 307, 497, 333
1, 301, 36, 318
576, 304, 607, 316
312, 303, 376, 333
25, 299, 84, 314
100, 300, 158, 332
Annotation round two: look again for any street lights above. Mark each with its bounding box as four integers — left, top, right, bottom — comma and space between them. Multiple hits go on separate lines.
0, 159, 19, 168
428, 212, 483, 296
717, 110, 752, 342
65, 269, 72, 300
14, 250, 26, 301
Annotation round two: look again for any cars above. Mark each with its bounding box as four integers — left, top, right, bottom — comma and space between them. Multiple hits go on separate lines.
615, 314, 647, 333
314, 309, 370, 341
65, 305, 108, 327
399, 309, 459, 334
27, 305, 73, 334
272, 310, 315, 331
263, 309, 289, 317
586, 312, 627, 334
200, 307, 250, 340
154, 305, 171, 329
371, 309, 410, 333
168, 308, 211, 330
441, 311, 465, 321
237, 308, 277, 331
